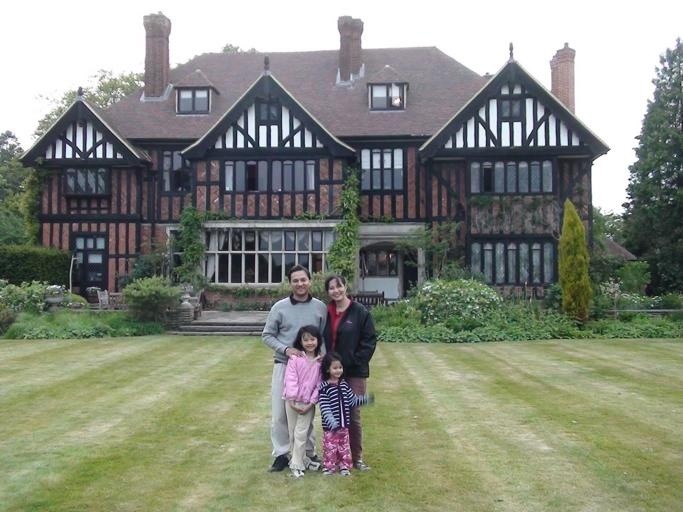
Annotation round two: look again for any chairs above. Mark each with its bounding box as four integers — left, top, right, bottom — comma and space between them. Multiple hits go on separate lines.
96, 289, 115, 310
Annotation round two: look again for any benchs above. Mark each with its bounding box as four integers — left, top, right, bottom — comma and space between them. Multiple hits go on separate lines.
346, 290, 385, 309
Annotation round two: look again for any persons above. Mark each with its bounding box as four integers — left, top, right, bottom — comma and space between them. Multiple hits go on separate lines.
260, 264, 328, 474
321, 273, 377, 474
282, 324, 324, 476
315, 351, 376, 478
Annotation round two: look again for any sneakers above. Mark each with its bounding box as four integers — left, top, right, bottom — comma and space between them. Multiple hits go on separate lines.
322, 469, 334, 476
307, 461, 321, 471
353, 461, 370, 472
267, 455, 291, 472
310, 455, 322, 462
291, 469, 305, 477
339, 470, 351, 476
